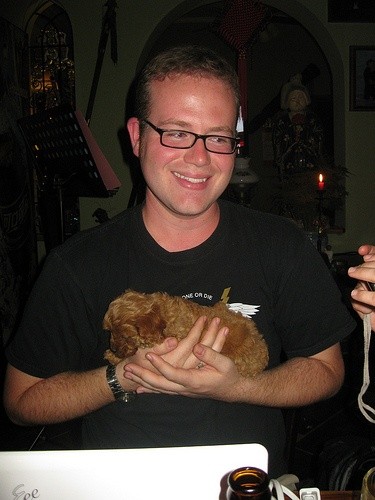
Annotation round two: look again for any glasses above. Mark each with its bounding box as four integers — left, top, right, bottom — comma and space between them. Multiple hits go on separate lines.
136, 116, 242, 155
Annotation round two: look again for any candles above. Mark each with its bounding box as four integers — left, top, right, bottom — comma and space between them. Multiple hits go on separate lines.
317, 174, 324, 193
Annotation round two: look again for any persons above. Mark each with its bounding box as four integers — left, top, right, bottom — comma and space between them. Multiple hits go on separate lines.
270, 70, 323, 168
348, 243, 375, 332
2, 46, 355, 478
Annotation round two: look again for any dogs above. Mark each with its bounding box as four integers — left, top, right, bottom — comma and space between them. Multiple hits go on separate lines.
102, 289, 269, 378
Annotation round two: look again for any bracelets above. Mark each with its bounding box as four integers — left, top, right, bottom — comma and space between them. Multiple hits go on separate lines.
106, 362, 124, 401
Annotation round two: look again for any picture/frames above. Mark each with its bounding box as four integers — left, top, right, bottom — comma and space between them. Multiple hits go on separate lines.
349, 45, 375, 112
